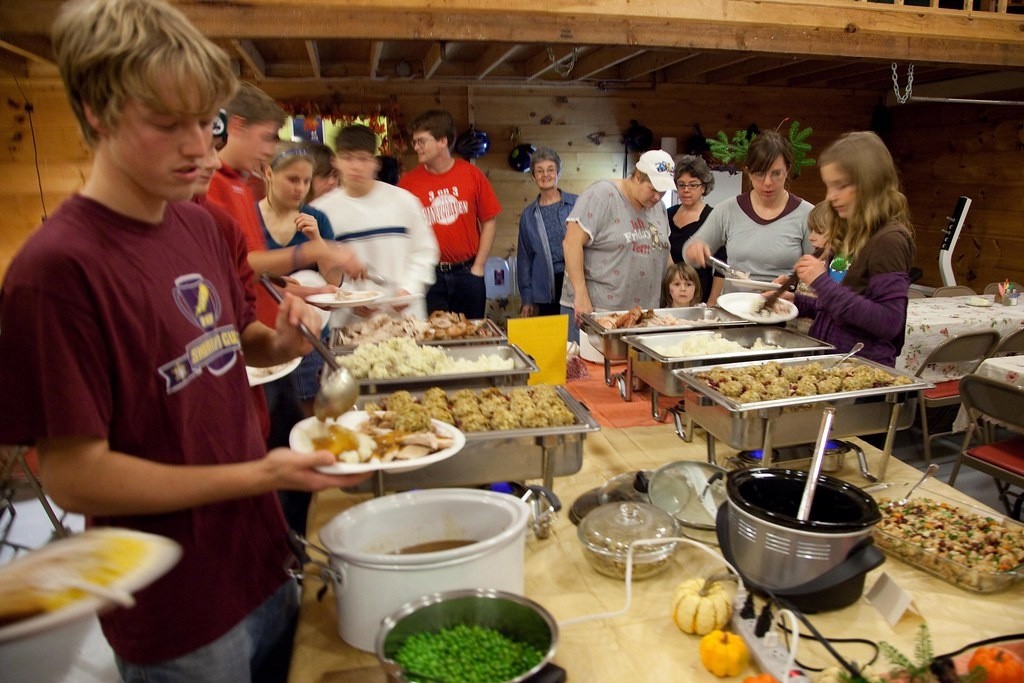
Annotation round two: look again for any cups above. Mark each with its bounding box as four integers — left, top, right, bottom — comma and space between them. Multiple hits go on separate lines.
1008, 298, 1017, 305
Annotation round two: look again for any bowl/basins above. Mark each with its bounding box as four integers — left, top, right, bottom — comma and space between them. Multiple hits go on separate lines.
376, 589, 559, 683
577, 501, 678, 579
869, 484, 1024, 594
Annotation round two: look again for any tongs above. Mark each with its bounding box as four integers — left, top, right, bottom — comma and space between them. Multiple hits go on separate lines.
707, 256, 743, 279
366, 271, 388, 287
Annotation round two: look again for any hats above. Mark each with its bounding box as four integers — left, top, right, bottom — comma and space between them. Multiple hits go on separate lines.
636, 149, 677, 192
211, 109, 228, 151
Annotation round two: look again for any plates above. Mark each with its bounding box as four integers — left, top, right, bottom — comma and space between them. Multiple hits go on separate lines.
384, 292, 426, 306
306, 291, 384, 308
717, 293, 799, 324
289, 411, 466, 473
0, 529, 182, 641
725, 278, 795, 290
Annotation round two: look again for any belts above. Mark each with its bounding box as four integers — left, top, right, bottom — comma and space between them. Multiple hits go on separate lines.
437, 259, 475, 273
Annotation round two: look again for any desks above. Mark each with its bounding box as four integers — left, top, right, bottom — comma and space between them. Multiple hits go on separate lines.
951, 355, 1024, 443
895, 292, 1024, 388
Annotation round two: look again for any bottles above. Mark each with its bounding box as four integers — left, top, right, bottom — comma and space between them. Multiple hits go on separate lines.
1004, 289, 1019, 306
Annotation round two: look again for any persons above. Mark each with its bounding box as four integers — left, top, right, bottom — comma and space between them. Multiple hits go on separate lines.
189, 82, 502, 539
559, 150, 728, 347
681, 130, 916, 368
0, 0, 375, 683
517, 147, 579, 318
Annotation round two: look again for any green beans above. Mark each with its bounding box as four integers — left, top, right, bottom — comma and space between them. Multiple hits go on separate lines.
879, 498, 1013, 560
393, 625, 545, 683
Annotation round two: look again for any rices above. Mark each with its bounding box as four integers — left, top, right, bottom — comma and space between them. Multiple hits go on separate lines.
665, 333, 783, 357
334, 336, 515, 380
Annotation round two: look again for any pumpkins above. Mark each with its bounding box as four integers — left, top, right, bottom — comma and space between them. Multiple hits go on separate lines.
672, 573, 781, 683
968, 646, 1024, 683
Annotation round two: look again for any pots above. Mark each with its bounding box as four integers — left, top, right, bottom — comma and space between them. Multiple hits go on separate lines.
290, 487, 562, 653
726, 464, 883, 593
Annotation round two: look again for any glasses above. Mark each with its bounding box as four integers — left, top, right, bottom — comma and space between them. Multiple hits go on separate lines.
676, 183, 704, 189
756, 166, 787, 178
410, 136, 430, 147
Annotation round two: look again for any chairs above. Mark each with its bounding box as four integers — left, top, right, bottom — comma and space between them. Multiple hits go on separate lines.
984, 326, 1024, 443
914, 329, 1001, 467
948, 374, 1024, 523
932, 286, 977, 297
908, 289, 927, 299
983, 282, 1024, 295
